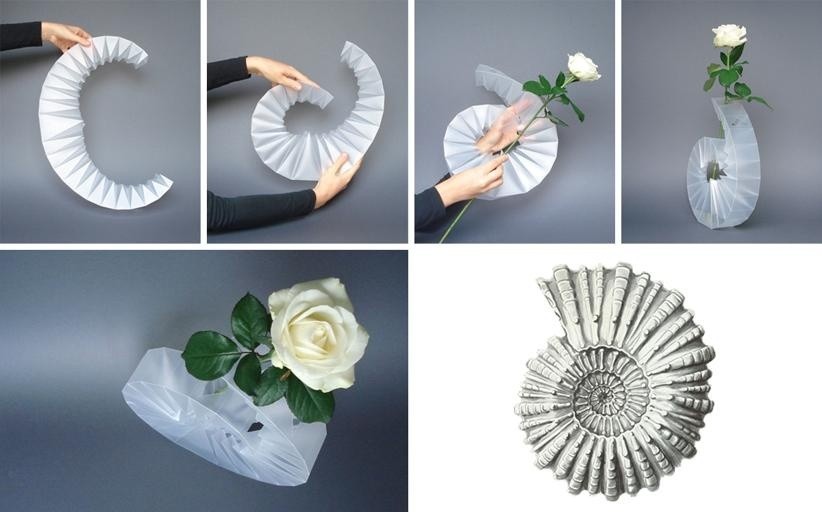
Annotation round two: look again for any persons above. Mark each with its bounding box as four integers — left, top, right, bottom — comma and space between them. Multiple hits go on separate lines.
0, 20, 94, 54
414, 98, 544, 233
207, 52, 363, 234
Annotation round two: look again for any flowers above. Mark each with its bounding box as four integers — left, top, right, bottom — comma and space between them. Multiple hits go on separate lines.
698, 15, 774, 111
182, 274, 370, 424
435, 51, 604, 243
